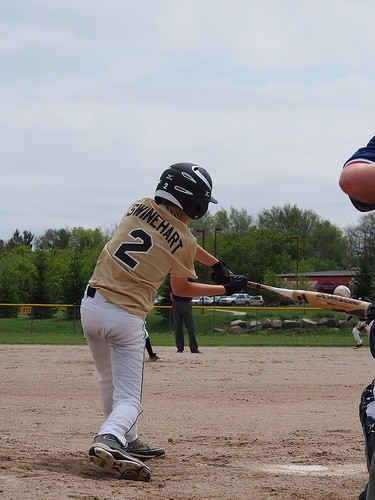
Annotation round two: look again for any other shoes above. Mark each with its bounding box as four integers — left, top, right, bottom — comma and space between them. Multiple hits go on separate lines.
177, 349, 184, 352
191, 349, 199, 353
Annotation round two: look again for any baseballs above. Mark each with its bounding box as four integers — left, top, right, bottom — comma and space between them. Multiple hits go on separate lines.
333, 285, 351, 298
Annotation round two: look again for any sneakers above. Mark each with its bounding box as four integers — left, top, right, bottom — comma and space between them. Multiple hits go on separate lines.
150, 356, 163, 362
353, 343, 363, 349
87, 434, 167, 482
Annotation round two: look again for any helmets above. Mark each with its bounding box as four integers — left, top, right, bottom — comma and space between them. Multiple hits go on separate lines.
334, 285, 351, 297
154, 163, 218, 220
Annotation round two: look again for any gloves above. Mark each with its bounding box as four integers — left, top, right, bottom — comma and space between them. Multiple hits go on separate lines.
222, 275, 248, 296
210, 260, 230, 285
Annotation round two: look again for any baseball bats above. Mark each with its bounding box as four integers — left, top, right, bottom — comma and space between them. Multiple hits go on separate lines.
246, 281, 372, 316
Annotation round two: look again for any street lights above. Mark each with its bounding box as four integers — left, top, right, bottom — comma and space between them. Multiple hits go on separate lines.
213, 228, 222, 306
290, 236, 299, 289
196, 230, 205, 314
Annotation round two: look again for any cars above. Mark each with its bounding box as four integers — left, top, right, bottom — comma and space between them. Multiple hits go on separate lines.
247, 295, 265, 306
219, 293, 251, 307
190, 296, 220, 306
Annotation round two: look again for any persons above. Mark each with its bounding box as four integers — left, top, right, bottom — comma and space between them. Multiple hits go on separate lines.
79, 163, 218, 480
342, 296, 375, 349
338, 143, 375, 212
141, 336, 161, 359
168, 269, 204, 356
